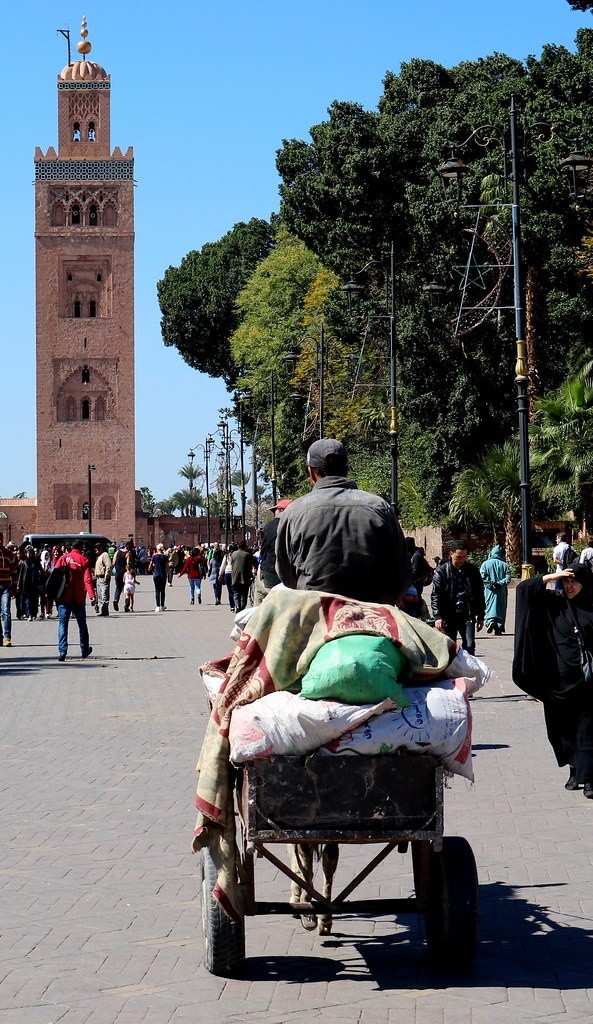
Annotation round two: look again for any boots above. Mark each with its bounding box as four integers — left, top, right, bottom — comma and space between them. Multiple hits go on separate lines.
98, 602, 109, 617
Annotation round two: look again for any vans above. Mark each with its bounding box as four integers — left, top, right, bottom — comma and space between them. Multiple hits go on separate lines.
22, 532, 116, 572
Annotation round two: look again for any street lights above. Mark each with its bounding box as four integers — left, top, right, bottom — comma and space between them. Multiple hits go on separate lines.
339, 241, 400, 522
281, 315, 325, 441
187, 369, 279, 547
87, 462, 97, 532
432, 91, 533, 579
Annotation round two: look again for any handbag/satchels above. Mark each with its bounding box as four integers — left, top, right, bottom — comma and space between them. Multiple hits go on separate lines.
46, 553, 68, 602
197, 556, 206, 576
579, 648, 593, 683
562, 544, 578, 569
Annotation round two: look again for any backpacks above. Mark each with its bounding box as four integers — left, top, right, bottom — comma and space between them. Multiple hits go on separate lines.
418, 555, 435, 586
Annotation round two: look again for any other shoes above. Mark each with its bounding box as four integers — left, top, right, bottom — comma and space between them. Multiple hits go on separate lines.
154, 607, 161, 612
494, 630, 502, 635
565, 766, 582, 791
124, 607, 130, 612
582, 782, 593, 799
59, 655, 66, 661
82, 647, 93, 658
486, 621, 495, 633
113, 601, 119, 611
161, 606, 167, 611
5, 637, 12, 647
0, 635, 3, 646
16, 612, 77, 622
190, 594, 246, 614
94, 604, 99, 613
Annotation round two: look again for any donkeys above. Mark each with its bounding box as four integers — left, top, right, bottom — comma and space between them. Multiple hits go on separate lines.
289, 841, 340, 936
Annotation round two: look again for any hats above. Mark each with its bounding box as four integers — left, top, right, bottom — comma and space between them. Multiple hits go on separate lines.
126, 541, 136, 548
269, 499, 292, 514
307, 436, 347, 468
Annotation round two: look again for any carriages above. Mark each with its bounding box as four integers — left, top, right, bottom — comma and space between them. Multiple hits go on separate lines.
199, 751, 480, 973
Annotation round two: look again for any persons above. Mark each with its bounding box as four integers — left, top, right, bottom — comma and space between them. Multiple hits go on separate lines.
252, 499, 293, 608
579, 541, 593, 572
405, 536, 433, 595
512, 564, 593, 798
553, 532, 576, 591
431, 556, 444, 567
432, 540, 486, 656
480, 545, 511, 634
5, 538, 272, 621
55, 542, 96, 661
0, 532, 19, 646
275, 438, 414, 606
